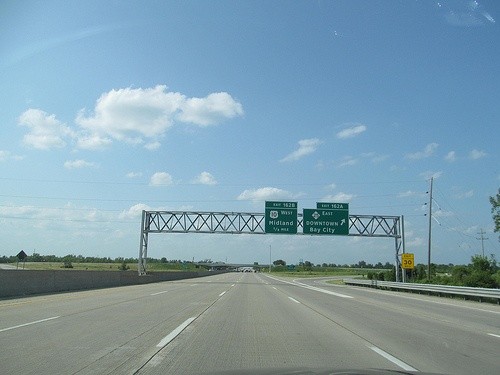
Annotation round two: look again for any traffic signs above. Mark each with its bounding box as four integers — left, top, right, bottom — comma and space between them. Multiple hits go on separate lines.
302, 208, 349, 235
264, 200, 297, 234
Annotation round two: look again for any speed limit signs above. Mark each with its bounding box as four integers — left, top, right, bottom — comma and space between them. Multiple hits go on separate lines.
402, 253, 414, 269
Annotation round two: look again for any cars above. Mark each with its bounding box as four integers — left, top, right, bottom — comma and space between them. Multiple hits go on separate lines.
209, 269, 214, 272
241, 267, 256, 272
